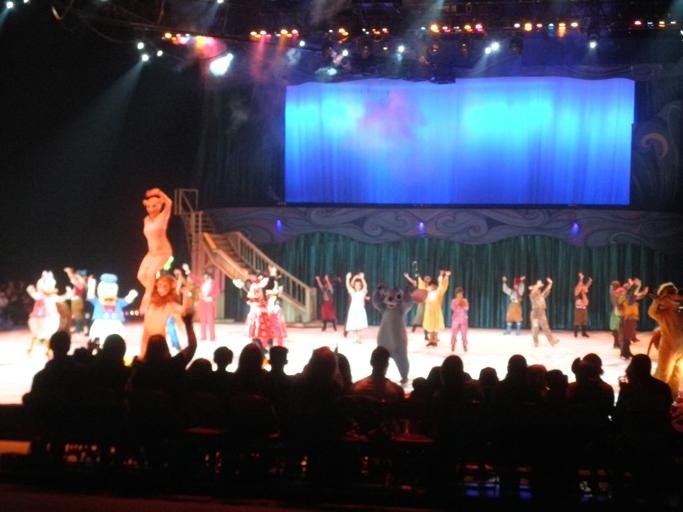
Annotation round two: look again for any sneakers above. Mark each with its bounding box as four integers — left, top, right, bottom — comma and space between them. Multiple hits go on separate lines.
412, 331, 471, 354
321, 328, 364, 345
504, 331, 640, 360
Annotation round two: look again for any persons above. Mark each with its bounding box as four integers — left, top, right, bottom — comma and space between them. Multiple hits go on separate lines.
136, 187, 172, 315
3, 262, 681, 510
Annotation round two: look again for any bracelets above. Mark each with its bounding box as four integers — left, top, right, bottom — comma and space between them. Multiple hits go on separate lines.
156, 189, 161, 197
